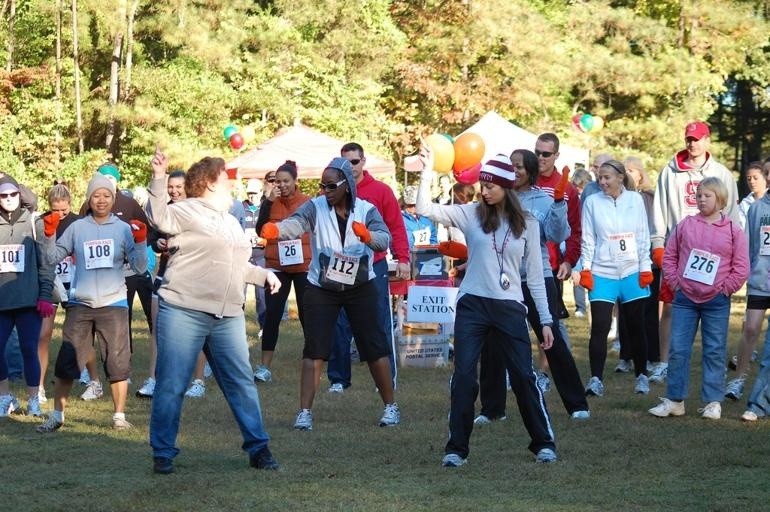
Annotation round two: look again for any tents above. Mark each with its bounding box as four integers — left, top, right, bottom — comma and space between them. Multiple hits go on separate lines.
222, 120, 394, 197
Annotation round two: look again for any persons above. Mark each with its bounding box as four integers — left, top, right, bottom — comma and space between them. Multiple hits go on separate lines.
0, 142, 410, 475
395, 120, 769, 475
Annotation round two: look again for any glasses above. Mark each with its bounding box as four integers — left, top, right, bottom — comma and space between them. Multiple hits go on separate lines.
348, 158, 362, 166
319, 179, 347, 190
267, 176, 276, 183
0, 191, 19, 199
536, 150, 556, 157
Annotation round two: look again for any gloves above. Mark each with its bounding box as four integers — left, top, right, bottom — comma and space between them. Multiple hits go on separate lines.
130, 220, 147, 243
353, 220, 370, 244
37, 300, 55, 318
44, 212, 60, 238
262, 220, 279, 240
579, 270, 593, 291
652, 247, 665, 268
640, 271, 653, 288
554, 165, 570, 200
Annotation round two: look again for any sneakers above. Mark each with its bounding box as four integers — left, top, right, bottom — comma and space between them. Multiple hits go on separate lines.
537, 372, 552, 394
185, 379, 206, 398
81, 381, 103, 401
253, 366, 272, 383
572, 410, 591, 419
379, 403, 400, 427
633, 373, 649, 394
648, 397, 685, 417
742, 411, 757, 421
729, 356, 737, 371
585, 376, 604, 396
293, 408, 313, 431
750, 351, 758, 362
36, 414, 64, 432
648, 363, 668, 383
204, 362, 214, 380
328, 383, 344, 393
112, 416, 133, 430
724, 378, 744, 401
536, 449, 556, 463
79, 368, 90, 385
135, 377, 156, 398
2, 393, 20, 417
26, 395, 42, 417
38, 386, 47, 403
610, 340, 621, 354
474, 415, 506, 424
697, 402, 721, 419
646, 361, 658, 373
615, 359, 635, 372
506, 369, 511, 392
247, 445, 280, 471
442, 454, 467, 466
151, 449, 175, 475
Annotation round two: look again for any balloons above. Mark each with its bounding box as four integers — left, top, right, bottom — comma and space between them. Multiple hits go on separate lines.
223, 123, 255, 149
574, 113, 581, 126
590, 116, 604, 135
579, 115, 595, 132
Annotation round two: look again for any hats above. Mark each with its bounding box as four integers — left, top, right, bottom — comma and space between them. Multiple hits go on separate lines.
684, 122, 710, 140
87, 172, 115, 205
246, 179, 262, 193
479, 154, 516, 189
403, 186, 418, 204
97, 163, 121, 182
